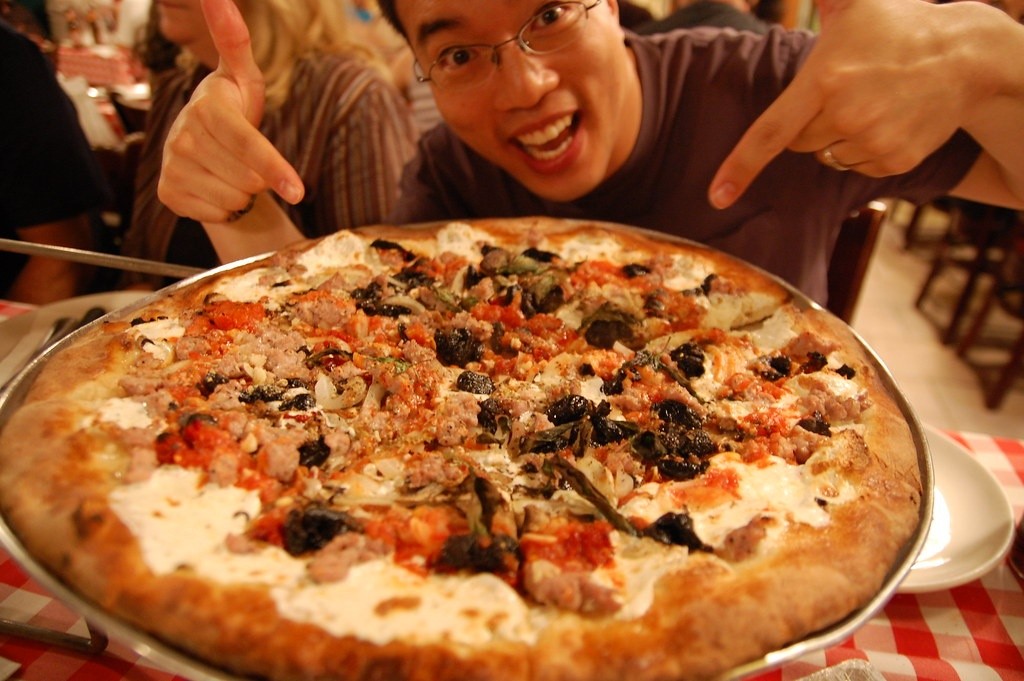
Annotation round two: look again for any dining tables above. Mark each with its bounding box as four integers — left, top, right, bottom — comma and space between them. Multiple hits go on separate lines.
1, 297, 1024, 681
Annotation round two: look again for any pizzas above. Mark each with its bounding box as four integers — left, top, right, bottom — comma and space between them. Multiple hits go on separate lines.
0, 214, 924, 681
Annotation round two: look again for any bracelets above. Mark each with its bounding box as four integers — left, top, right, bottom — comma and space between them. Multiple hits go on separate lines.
227, 195, 256, 222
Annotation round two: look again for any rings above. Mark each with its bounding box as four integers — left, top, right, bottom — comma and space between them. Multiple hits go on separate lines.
825, 149, 852, 171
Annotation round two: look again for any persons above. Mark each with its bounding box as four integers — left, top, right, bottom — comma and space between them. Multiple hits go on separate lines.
156, 0, 1024, 310
0, 25, 114, 306
119, 1, 423, 292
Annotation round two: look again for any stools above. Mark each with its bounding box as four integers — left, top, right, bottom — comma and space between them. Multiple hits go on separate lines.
888, 194, 1024, 412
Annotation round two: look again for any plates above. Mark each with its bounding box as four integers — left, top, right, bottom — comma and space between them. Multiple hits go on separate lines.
0, 219, 934, 680
890, 424, 1015, 593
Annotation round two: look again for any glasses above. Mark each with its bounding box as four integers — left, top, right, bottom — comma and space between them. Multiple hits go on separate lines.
412, 0, 602, 83
